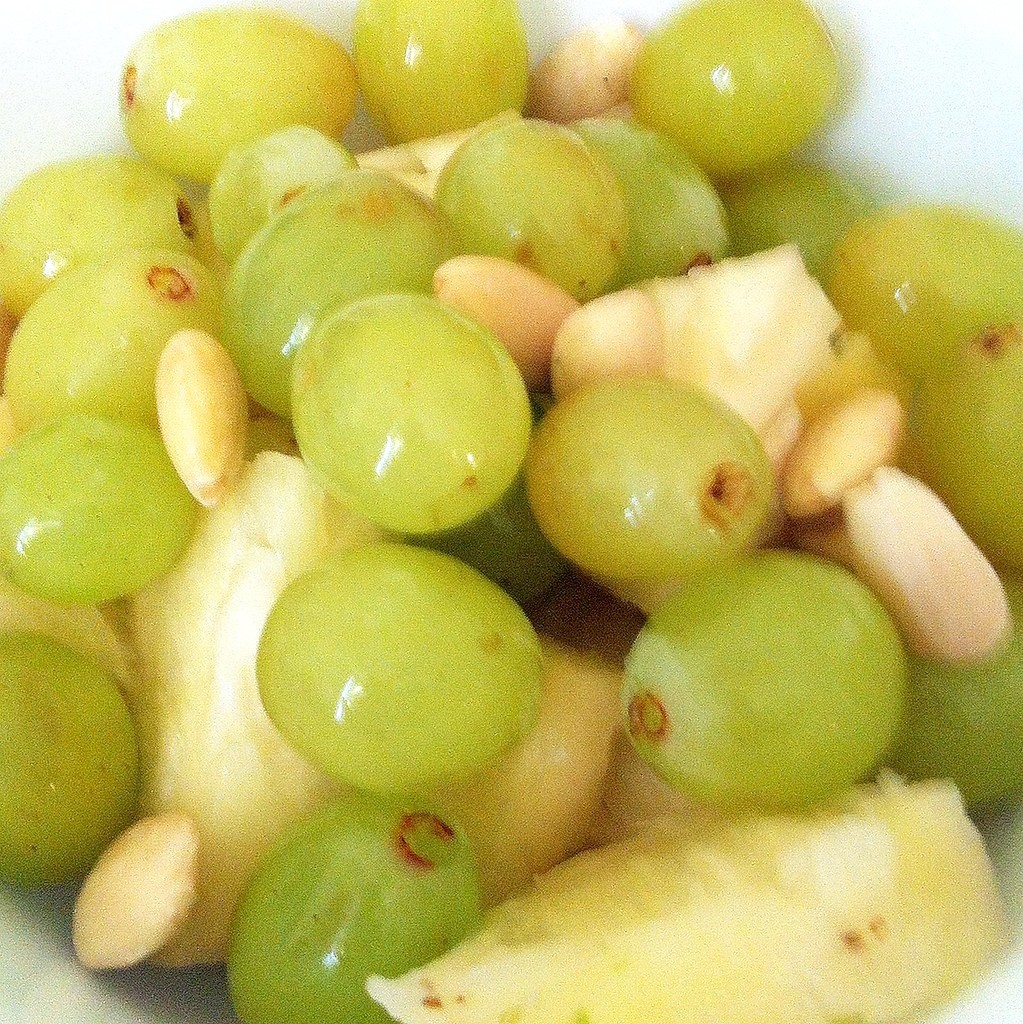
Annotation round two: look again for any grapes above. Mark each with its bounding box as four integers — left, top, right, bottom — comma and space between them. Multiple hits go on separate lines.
1, 0, 1022, 1023
